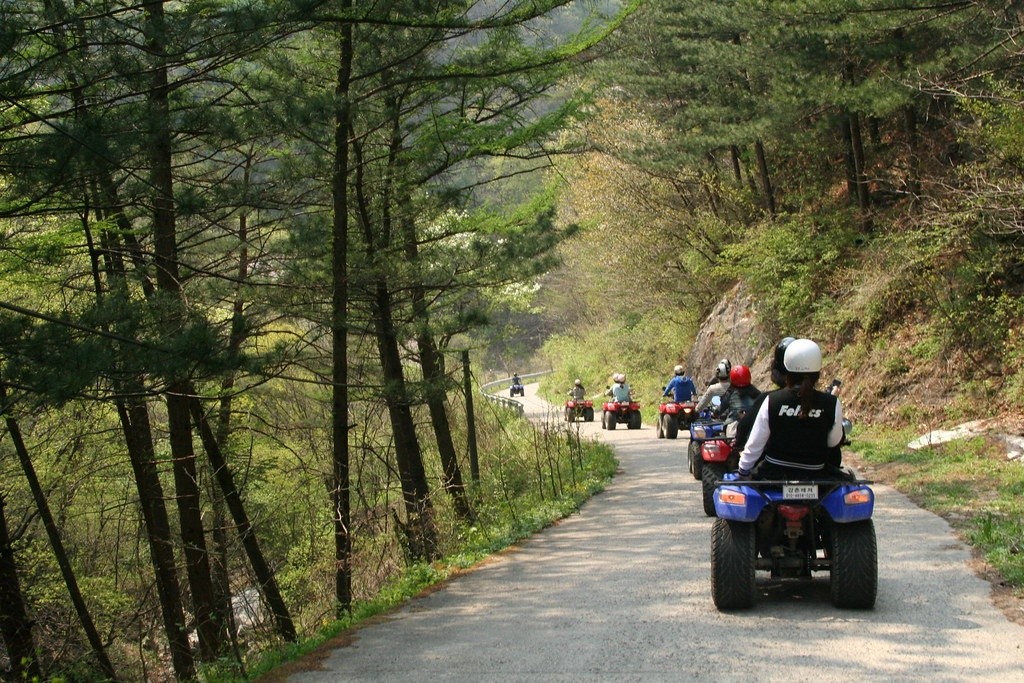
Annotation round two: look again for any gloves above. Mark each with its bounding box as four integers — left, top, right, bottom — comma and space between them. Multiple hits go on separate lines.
731, 467, 751, 477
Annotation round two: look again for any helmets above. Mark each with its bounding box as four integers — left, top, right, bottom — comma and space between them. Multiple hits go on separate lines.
514, 373, 517, 376
674, 365, 685, 374
775, 337, 797, 371
618, 374, 625, 382
730, 366, 751, 387
784, 339, 821, 372
720, 359, 731, 371
613, 373, 620, 381
575, 379, 581, 385
716, 363, 729, 378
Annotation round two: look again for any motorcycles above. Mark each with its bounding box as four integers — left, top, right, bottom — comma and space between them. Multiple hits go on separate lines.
688, 399, 724, 481
510, 378, 524, 397
601, 384, 642, 430
703, 405, 740, 517
564, 387, 594, 422
657, 386, 700, 439
709, 406, 878, 614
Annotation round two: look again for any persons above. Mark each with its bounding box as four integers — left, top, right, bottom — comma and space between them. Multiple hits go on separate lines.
512, 373, 520, 385
735, 337, 848, 481
605, 374, 633, 402
568, 379, 586, 401
695, 359, 761, 438
663, 365, 696, 403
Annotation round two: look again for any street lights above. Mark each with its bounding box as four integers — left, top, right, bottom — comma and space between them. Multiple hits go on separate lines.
529, 333, 541, 348
